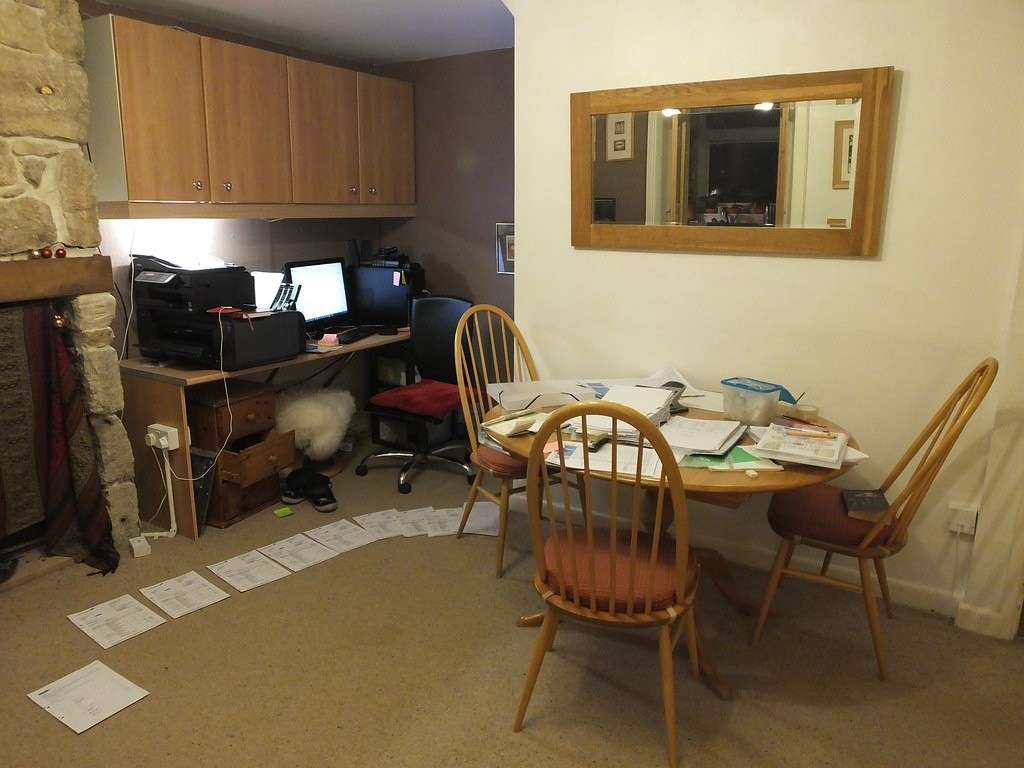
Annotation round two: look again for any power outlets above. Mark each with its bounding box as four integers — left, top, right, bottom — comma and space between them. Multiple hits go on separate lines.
947, 505, 977, 535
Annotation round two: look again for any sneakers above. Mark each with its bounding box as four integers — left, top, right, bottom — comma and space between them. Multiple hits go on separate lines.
307, 472, 338, 511
282, 467, 308, 503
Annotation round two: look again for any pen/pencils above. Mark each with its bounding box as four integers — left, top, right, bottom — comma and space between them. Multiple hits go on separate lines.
786, 431, 836, 439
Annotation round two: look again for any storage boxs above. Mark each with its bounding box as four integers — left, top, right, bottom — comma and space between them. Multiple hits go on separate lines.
721, 376, 783, 426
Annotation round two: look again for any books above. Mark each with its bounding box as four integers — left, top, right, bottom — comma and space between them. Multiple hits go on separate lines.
842, 489, 892, 526
209, 307, 271, 319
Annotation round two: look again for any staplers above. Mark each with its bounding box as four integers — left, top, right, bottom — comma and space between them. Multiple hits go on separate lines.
587, 432, 608, 453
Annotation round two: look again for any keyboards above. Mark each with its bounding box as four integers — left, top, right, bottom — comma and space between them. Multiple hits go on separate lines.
337, 326, 381, 344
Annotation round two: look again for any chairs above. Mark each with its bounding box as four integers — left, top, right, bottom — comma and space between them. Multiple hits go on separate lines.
454, 303, 586, 577
514, 399, 699, 768
750, 358, 999, 680
355, 296, 485, 494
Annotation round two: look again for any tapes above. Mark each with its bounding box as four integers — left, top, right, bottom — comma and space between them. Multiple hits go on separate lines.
796, 405, 819, 422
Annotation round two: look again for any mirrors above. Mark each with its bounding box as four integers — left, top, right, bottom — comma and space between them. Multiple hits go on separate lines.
570, 65, 895, 258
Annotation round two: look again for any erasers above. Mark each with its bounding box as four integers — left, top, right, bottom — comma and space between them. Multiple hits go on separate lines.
746, 469, 759, 478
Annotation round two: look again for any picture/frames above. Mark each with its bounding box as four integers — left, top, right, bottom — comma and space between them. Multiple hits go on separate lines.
833, 120, 854, 189
605, 111, 635, 161
494, 222, 515, 275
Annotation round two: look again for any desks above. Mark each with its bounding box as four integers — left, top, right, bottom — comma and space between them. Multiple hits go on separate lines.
117, 326, 427, 541
481, 393, 859, 700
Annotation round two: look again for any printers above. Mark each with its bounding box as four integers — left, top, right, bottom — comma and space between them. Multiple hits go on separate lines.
129, 251, 307, 372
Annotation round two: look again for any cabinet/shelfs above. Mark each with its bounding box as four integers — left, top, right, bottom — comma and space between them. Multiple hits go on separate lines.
185, 378, 296, 529
83, 14, 417, 218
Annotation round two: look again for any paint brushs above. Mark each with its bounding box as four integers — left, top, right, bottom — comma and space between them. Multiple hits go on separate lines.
793, 387, 809, 405
783, 414, 828, 428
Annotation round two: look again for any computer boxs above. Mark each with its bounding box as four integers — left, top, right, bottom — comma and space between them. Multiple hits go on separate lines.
347, 264, 425, 328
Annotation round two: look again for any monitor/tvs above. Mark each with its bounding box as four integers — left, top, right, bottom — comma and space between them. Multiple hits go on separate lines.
285, 257, 350, 339
594, 198, 616, 222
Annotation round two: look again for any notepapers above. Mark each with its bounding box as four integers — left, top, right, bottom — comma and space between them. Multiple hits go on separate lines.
318, 334, 339, 347
